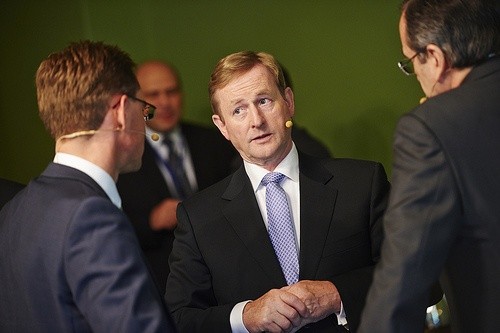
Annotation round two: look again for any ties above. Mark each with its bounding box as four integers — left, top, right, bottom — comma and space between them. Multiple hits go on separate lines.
261, 172, 299, 287
162, 136, 192, 200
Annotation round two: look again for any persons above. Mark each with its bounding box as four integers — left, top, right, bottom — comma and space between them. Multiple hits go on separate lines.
0, 39, 169, 333
163, 50, 392, 332
115, 59, 244, 260
355, 0, 500, 333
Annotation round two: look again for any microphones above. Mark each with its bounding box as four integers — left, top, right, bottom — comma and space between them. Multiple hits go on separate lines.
56, 129, 159, 140
418, 67, 451, 105
285, 120, 293, 128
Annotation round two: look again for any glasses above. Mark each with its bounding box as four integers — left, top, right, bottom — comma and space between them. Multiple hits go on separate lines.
398, 47, 425, 75
112, 95, 156, 121
